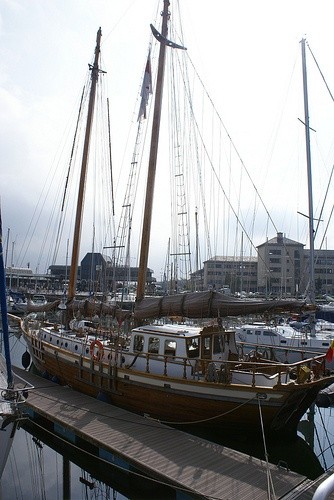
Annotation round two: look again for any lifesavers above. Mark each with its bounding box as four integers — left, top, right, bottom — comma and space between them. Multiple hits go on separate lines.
90, 340, 105, 361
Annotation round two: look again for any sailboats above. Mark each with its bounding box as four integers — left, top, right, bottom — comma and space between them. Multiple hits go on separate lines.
0, 0, 334, 433
